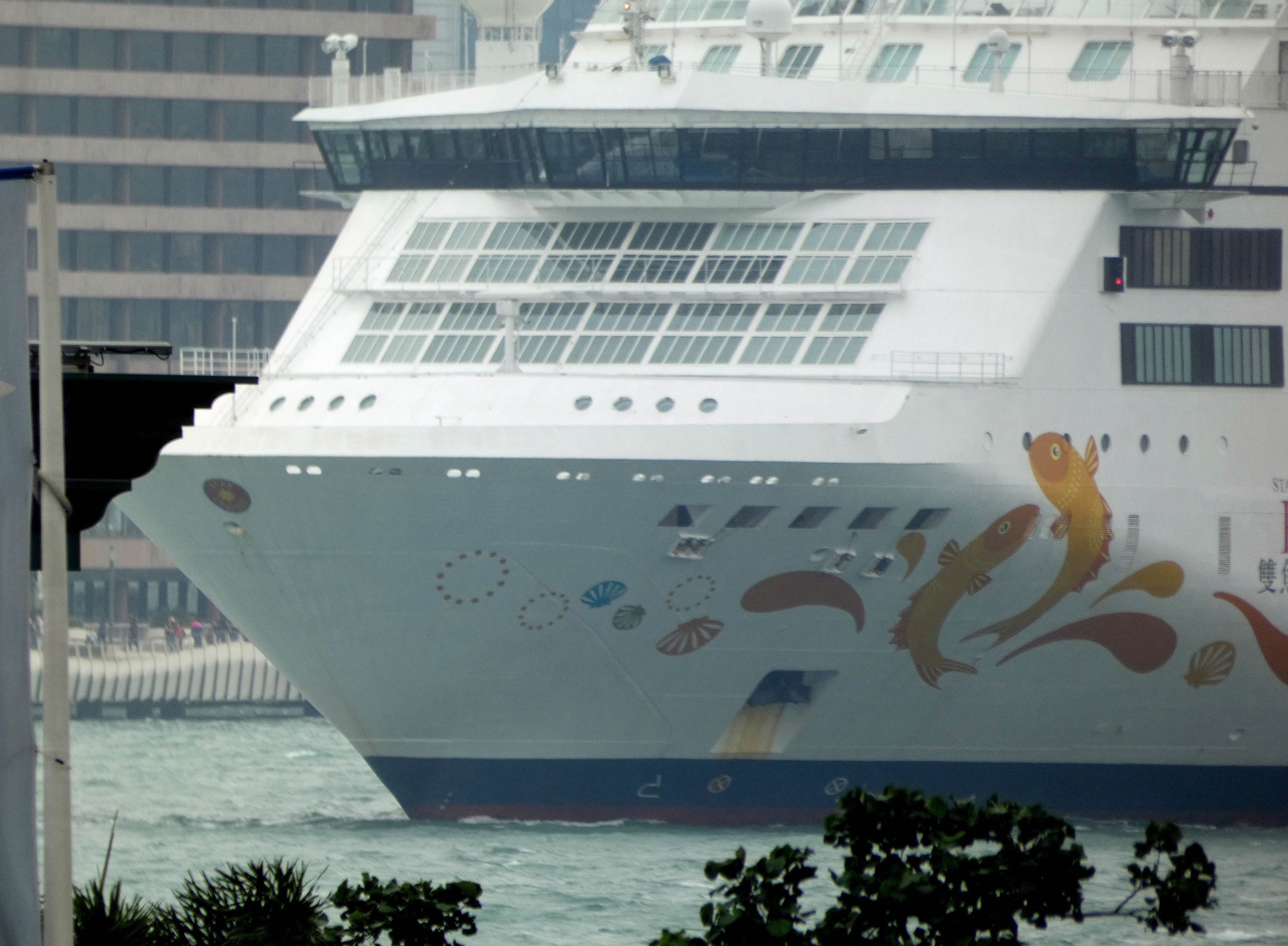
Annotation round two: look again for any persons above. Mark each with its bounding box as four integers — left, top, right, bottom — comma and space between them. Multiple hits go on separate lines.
26, 608, 234, 652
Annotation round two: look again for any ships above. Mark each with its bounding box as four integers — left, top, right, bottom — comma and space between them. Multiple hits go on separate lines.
119, 0, 1288, 833
